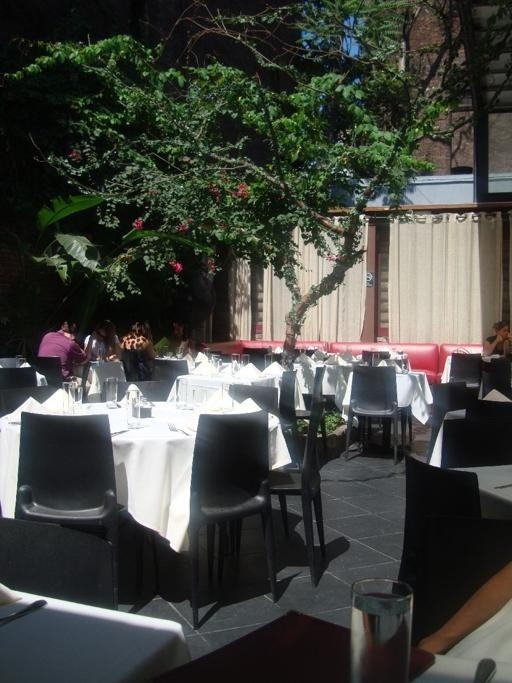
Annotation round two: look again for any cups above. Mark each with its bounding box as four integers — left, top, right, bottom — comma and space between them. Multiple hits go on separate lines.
242, 354, 249, 367
264, 354, 272, 369
126, 390, 140, 429
68, 387, 82, 415
177, 347, 183, 359
349, 577, 414, 683
193, 389, 204, 415
104, 377, 118, 409
232, 354, 240, 371
222, 384, 233, 410
176, 378, 188, 411
204, 348, 210, 359
62, 382, 76, 414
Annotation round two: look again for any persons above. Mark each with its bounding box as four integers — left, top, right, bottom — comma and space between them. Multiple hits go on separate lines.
37, 321, 96, 381
120, 321, 156, 359
171, 319, 196, 357
484, 322, 512, 357
410, 556, 512, 683
83, 318, 120, 362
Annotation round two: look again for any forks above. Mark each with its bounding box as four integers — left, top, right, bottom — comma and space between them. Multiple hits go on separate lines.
168, 423, 190, 436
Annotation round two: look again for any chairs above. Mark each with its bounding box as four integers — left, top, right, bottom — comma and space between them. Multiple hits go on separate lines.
30, 356, 64, 386
392, 454, 482, 644
15, 411, 118, 549
0, 367, 38, 386
450, 353, 482, 383
269, 397, 327, 588
362, 350, 390, 366
100, 381, 173, 402
121, 348, 151, 381
297, 365, 327, 454
427, 381, 468, 464
343, 369, 403, 465
1, 386, 62, 417
279, 369, 303, 472
440, 418, 511, 467
228, 383, 278, 416
481, 361, 511, 402
0, 517, 115, 611
465, 399, 512, 417
135, 409, 279, 630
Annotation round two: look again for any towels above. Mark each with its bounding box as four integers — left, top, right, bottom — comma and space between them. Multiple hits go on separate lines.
233, 398, 262, 414
42, 389, 72, 413
192, 361, 218, 376
8, 396, 50, 423
120, 384, 143, 403
236, 363, 261, 379
